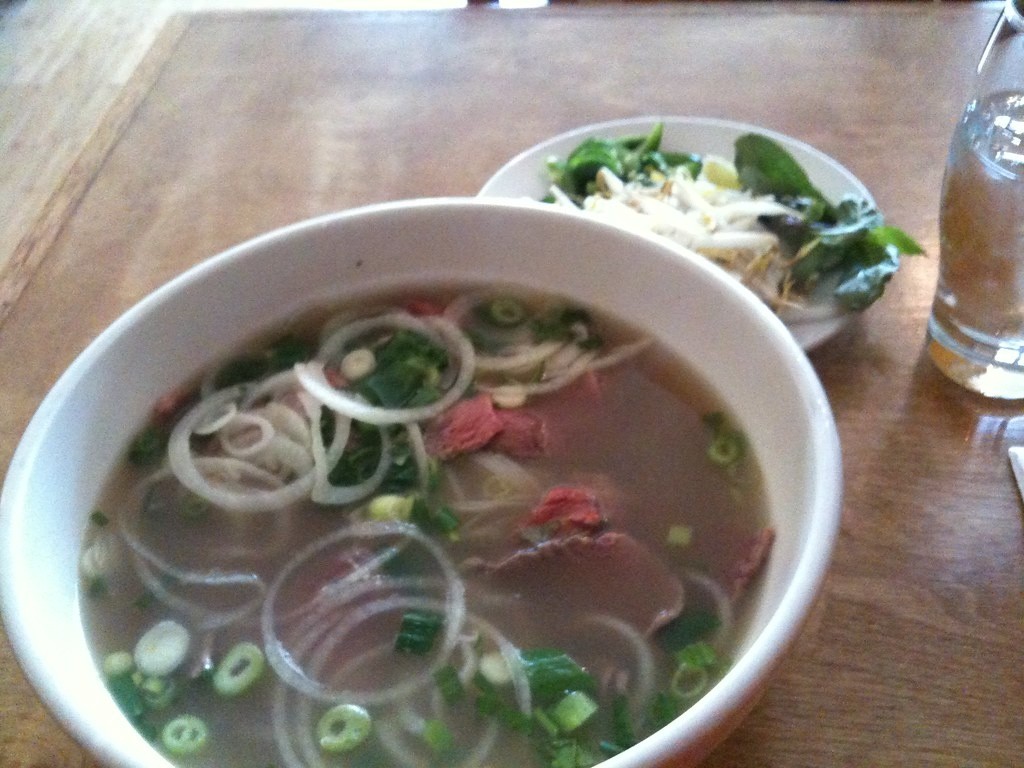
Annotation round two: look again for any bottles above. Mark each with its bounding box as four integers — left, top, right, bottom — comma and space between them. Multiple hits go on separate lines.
925, 0, 1024, 401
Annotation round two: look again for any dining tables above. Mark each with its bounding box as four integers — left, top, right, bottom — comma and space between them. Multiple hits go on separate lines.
0, 7, 1023, 767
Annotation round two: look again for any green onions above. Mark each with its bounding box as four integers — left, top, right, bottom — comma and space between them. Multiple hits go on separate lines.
78, 294, 747, 768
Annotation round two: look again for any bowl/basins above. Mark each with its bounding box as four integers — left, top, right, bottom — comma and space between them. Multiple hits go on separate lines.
0, 197, 845, 768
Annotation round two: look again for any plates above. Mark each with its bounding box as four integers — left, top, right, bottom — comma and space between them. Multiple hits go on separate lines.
474, 114, 882, 352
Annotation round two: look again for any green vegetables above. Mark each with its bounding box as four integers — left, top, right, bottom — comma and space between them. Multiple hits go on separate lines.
541, 121, 932, 313
